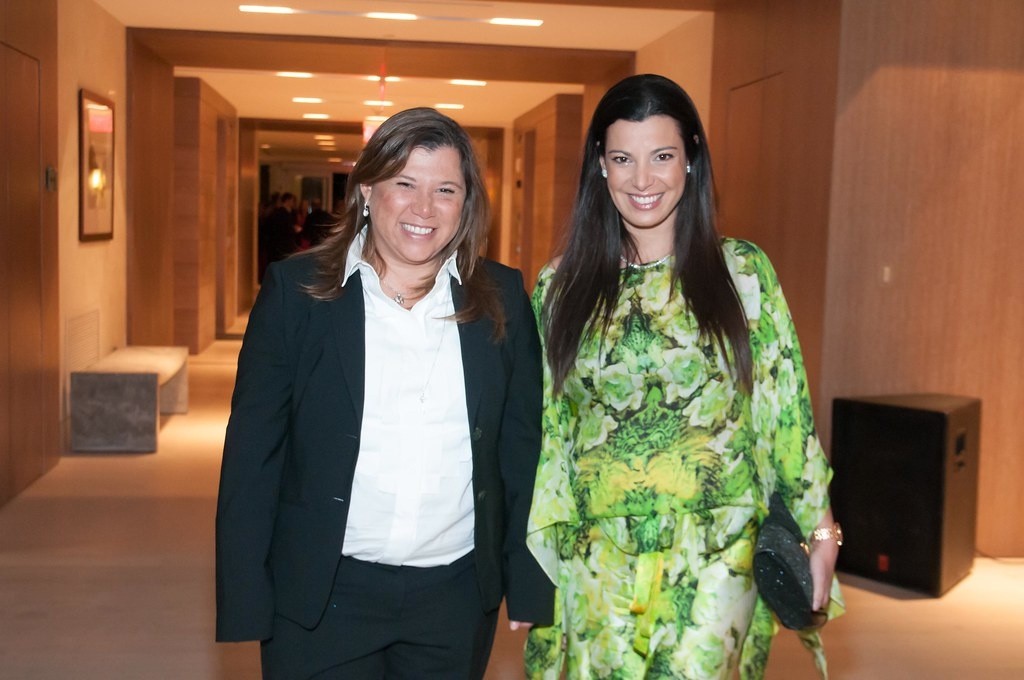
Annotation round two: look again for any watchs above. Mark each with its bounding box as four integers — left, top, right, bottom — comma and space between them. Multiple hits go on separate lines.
811, 523, 843, 547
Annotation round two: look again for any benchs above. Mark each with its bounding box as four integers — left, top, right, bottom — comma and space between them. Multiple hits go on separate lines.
70, 345, 190, 455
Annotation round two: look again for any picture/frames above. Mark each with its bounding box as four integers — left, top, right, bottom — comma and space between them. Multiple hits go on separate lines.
78, 88, 116, 243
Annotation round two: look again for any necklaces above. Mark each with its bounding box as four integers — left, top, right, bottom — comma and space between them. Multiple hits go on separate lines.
369, 256, 442, 304
618, 253, 673, 269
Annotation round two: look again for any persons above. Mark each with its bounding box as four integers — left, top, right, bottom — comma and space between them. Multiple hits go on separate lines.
257, 192, 338, 283
214, 106, 557, 680
522, 71, 846, 680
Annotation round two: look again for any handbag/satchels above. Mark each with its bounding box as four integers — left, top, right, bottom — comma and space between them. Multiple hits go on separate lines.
752, 492, 815, 632
